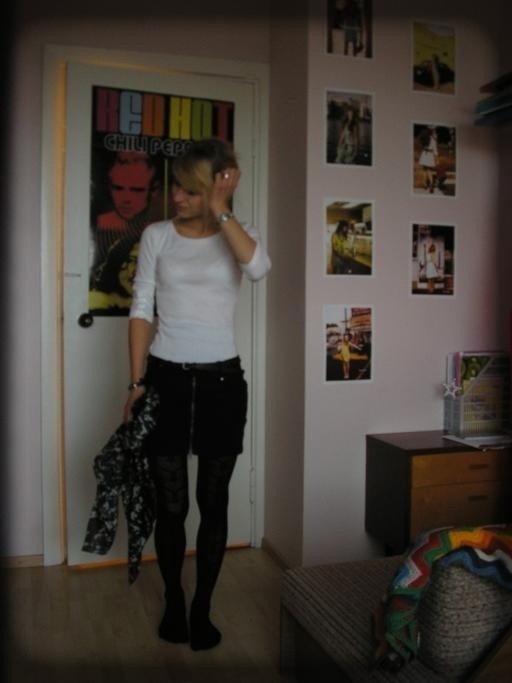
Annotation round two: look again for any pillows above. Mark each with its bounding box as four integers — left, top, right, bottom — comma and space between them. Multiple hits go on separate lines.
416, 561, 512, 682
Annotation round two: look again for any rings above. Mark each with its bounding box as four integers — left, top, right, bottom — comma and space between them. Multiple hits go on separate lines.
223, 173, 229, 178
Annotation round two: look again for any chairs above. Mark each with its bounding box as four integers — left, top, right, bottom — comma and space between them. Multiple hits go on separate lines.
272, 553, 512, 683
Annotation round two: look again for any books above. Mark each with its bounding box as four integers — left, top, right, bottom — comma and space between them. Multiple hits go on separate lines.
451, 351, 512, 438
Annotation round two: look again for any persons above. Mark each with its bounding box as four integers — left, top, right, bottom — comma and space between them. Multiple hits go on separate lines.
122, 136, 273, 652
90, 236, 141, 309
465, 356, 481, 378
430, 54, 442, 91
337, 332, 363, 378
340, 0, 365, 56
336, 106, 357, 160
330, 219, 360, 273
420, 242, 440, 293
96, 147, 163, 258
417, 124, 441, 193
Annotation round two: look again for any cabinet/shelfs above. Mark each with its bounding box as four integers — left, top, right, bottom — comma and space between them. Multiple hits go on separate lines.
365, 429, 512, 557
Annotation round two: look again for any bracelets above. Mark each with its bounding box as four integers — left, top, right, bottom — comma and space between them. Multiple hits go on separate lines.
127, 379, 145, 390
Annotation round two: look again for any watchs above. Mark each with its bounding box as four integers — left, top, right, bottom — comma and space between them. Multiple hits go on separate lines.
217, 211, 235, 225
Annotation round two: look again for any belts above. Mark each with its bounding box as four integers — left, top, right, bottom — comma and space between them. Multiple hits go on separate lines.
147, 352, 244, 376
